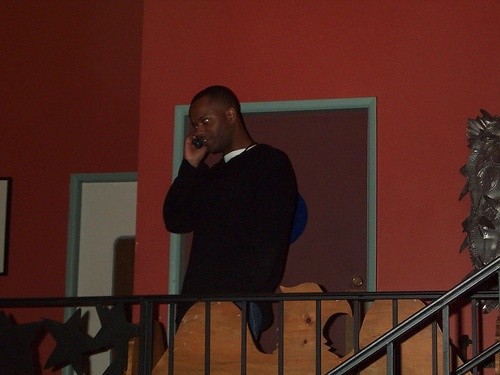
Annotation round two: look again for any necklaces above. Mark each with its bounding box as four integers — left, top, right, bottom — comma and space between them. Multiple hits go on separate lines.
242, 141, 256, 154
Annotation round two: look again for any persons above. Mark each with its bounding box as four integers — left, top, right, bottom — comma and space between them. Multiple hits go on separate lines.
160, 84, 299, 353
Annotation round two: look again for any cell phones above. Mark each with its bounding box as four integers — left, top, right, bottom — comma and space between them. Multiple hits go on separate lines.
193, 135, 204, 148
